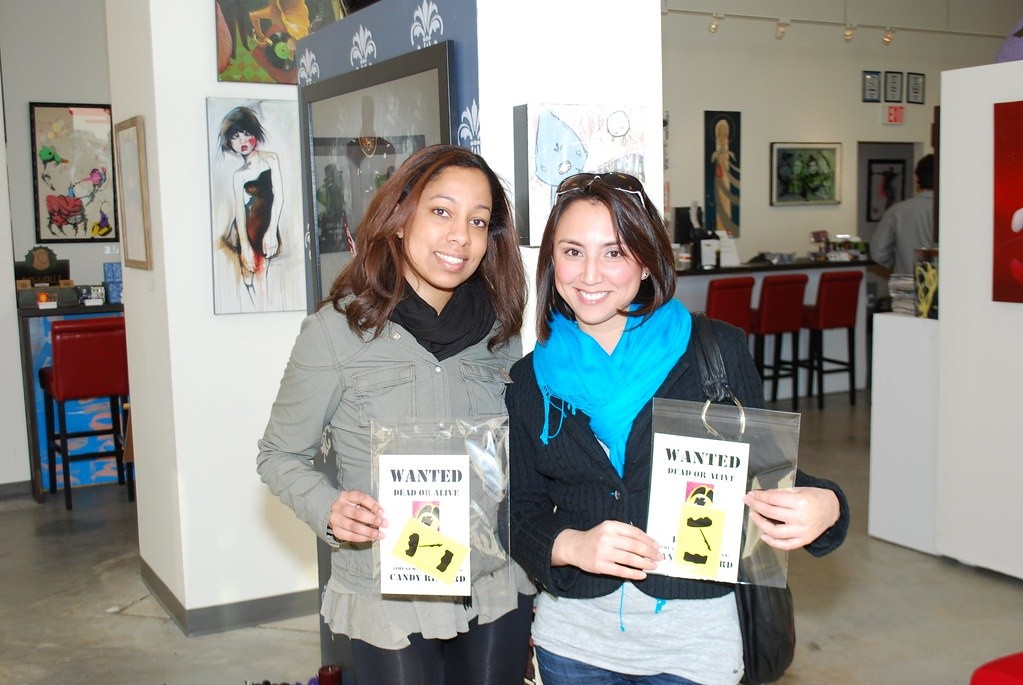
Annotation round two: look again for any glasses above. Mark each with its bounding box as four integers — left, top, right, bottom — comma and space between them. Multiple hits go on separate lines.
555, 172, 645, 210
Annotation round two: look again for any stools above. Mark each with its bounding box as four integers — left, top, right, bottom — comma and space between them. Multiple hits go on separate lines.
39, 315, 134, 509
750, 274, 809, 412
803, 269, 865, 410
704, 277, 756, 342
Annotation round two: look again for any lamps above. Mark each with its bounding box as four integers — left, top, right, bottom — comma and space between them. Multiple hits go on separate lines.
882, 27, 896, 44
708, 13, 727, 32
774, 17, 791, 39
842, 23, 862, 44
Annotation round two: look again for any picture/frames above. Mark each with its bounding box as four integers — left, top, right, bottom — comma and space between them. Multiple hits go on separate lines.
30, 102, 120, 245
114, 115, 155, 273
885, 72, 903, 103
862, 70, 881, 102
769, 142, 842, 207
907, 72, 925, 105
301, 41, 470, 318
866, 158, 906, 223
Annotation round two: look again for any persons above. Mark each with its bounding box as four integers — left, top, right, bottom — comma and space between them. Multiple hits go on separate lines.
255, 145, 528, 685
866, 155, 939, 277
495, 172, 849, 685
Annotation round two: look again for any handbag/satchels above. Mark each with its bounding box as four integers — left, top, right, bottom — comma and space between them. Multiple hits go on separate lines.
693, 310, 796, 685
